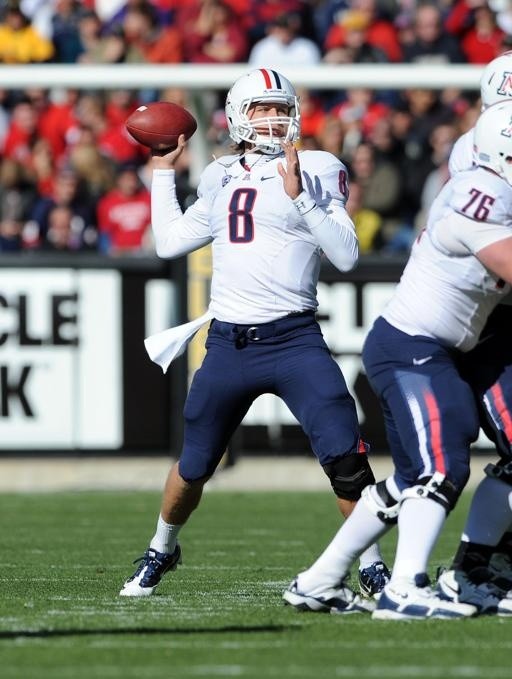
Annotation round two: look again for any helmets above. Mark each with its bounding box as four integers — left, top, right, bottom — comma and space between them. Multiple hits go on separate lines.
472, 99, 512, 185
225, 69, 301, 156
480, 51, 512, 107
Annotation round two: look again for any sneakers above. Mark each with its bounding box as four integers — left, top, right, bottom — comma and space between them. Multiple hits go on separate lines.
434, 568, 512, 617
281, 572, 377, 615
371, 573, 478, 620
120, 541, 182, 597
358, 561, 392, 600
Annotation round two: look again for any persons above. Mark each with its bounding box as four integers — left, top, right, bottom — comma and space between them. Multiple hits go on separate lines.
434, 54, 511, 617
117, 68, 395, 602
283, 101, 511, 621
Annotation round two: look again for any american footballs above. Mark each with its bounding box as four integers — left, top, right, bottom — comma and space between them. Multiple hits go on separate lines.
124, 102, 197, 151
